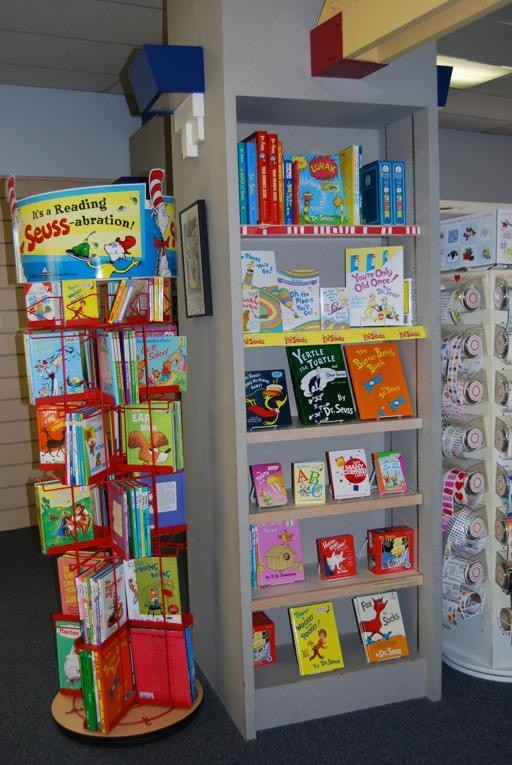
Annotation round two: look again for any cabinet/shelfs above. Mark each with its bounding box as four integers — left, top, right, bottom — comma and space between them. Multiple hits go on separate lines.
440, 268, 511, 680
169, 96, 442, 745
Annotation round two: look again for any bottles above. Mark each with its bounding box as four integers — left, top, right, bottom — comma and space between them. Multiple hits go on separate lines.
439, 280, 511, 637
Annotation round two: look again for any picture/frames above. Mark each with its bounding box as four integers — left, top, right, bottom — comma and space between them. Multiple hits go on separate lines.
178, 198, 215, 320
